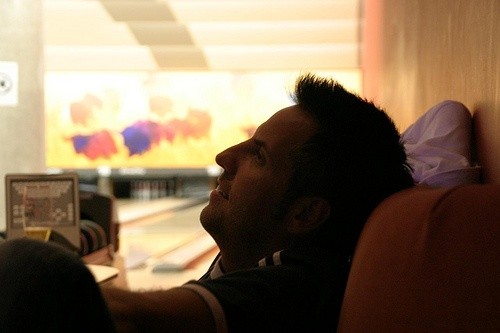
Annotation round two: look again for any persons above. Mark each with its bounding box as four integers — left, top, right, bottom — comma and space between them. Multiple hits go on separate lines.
1, 74, 417, 333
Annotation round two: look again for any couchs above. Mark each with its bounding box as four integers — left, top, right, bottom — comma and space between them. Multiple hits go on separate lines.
0, 189, 121, 272
